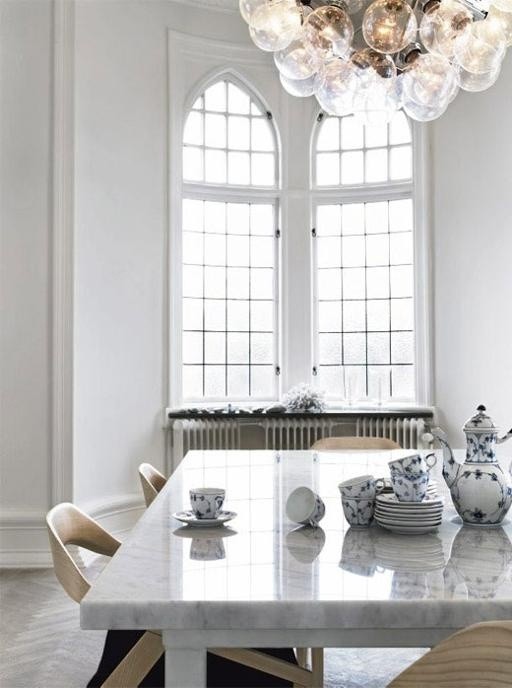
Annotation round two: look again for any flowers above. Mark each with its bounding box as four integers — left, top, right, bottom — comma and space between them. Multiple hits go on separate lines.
282, 385, 326, 412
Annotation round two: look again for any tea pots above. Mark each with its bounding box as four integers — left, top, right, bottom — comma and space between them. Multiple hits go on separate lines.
443, 525, 511, 600
434, 405, 512, 527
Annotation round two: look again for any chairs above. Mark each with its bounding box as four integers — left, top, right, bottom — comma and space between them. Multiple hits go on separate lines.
307, 435, 403, 451
137, 460, 167, 510
46, 503, 317, 687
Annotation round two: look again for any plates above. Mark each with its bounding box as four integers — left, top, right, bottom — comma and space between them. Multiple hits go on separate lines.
173, 526, 237, 539
173, 510, 237, 528
375, 496, 443, 534
373, 536, 444, 573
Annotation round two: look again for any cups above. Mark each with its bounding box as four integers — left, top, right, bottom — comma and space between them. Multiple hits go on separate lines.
285, 525, 325, 564
388, 453, 437, 473
189, 488, 225, 520
190, 538, 225, 560
337, 475, 386, 499
341, 497, 374, 531
391, 473, 429, 502
285, 486, 325, 528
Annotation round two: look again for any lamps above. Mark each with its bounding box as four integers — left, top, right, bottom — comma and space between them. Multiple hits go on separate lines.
235, 0, 512, 122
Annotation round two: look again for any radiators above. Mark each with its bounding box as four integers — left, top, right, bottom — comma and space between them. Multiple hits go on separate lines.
172, 416, 435, 471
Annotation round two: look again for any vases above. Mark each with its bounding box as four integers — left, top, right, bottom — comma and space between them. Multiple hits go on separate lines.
297, 400, 315, 412
